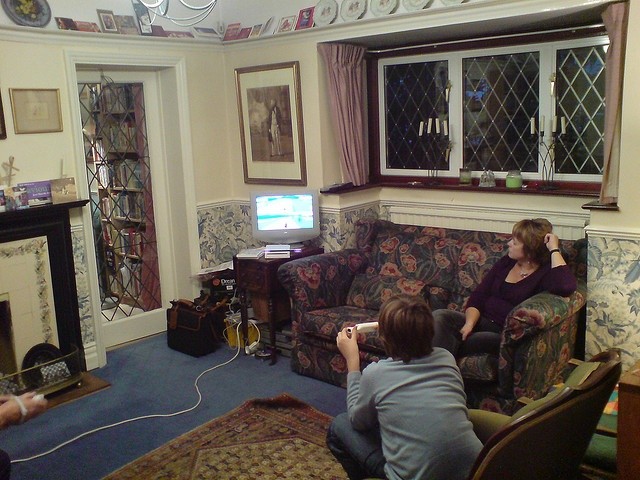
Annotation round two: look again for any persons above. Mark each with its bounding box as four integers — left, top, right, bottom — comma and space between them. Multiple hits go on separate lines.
0, 392, 48, 480
326, 293, 485, 480
266, 99, 285, 156
432, 218, 578, 352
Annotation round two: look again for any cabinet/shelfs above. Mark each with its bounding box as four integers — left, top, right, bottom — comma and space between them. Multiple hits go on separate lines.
88, 84, 148, 310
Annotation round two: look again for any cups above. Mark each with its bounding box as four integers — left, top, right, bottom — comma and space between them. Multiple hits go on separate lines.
459, 167, 471, 186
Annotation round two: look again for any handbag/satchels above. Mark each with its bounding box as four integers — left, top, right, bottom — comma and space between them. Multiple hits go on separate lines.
167, 294, 229, 358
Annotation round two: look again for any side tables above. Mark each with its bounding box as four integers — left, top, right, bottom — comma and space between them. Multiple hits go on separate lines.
232, 245, 323, 365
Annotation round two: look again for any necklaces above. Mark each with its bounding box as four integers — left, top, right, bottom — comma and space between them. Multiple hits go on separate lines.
517, 262, 538, 277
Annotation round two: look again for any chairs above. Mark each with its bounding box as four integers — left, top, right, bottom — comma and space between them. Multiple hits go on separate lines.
465, 348, 621, 479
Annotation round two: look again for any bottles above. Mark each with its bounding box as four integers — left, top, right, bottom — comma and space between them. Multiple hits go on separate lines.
506, 170, 523, 189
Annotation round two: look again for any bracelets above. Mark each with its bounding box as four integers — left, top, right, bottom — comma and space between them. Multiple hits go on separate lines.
549, 248, 561, 254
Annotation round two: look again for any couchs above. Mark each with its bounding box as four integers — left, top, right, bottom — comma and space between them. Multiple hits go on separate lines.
276, 218, 588, 415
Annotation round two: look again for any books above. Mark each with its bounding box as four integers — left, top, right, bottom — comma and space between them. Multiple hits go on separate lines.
108, 274, 118, 293
94, 139, 107, 162
101, 197, 110, 219
236, 248, 265, 260
111, 192, 144, 220
104, 84, 131, 111
97, 165, 112, 186
112, 227, 144, 256
108, 158, 141, 188
119, 262, 143, 298
104, 120, 137, 149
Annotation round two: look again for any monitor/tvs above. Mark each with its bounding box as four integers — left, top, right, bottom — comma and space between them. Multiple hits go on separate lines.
251, 188, 321, 249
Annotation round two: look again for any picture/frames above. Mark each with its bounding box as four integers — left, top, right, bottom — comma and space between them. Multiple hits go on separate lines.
234, 61, 307, 185
133, 3, 153, 36
259, 16, 274, 36
296, 7, 314, 28
0, 0, 51, 26
97, 9, 119, 32
0, 92, 7, 140
8, 87, 63, 133
249, 22, 263, 39
275, 16, 295, 32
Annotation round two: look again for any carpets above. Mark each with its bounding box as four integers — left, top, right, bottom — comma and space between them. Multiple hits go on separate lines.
100, 392, 349, 480
